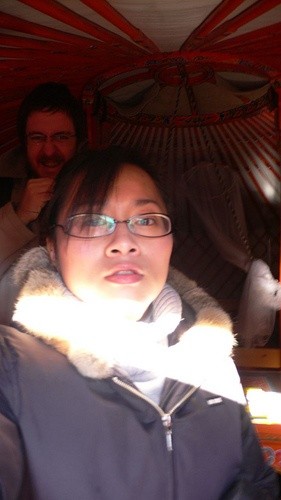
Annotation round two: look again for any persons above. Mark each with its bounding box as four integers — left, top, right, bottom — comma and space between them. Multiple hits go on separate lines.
0, 150, 281, 500
0, 81, 90, 283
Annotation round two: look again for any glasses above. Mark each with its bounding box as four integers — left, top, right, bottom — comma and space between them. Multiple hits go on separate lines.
49, 213, 175, 239
24, 132, 76, 146
